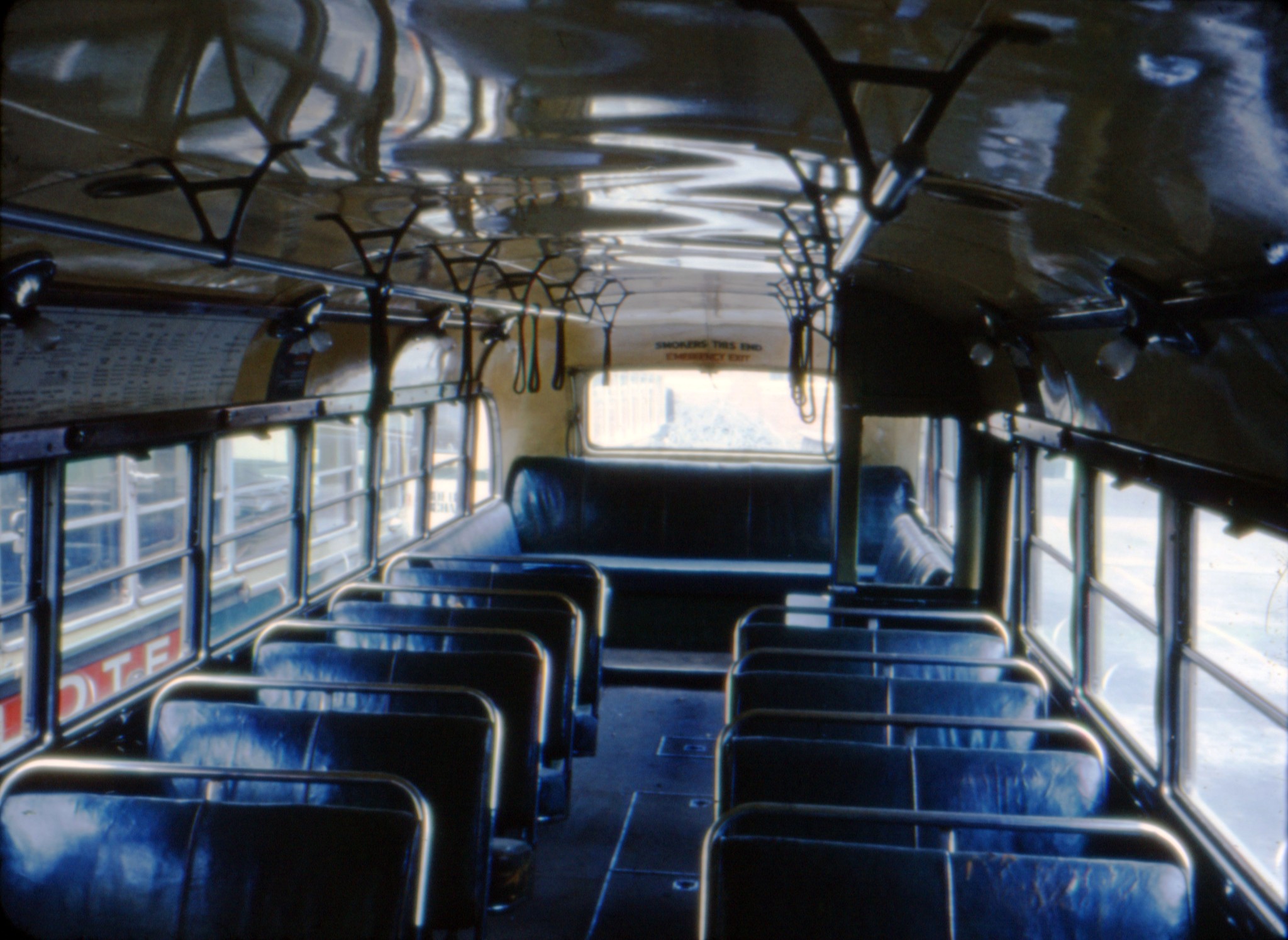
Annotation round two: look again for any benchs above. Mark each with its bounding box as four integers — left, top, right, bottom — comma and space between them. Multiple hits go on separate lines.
693, 599, 1202, 940
505, 457, 919, 595
0, 547, 605, 940
